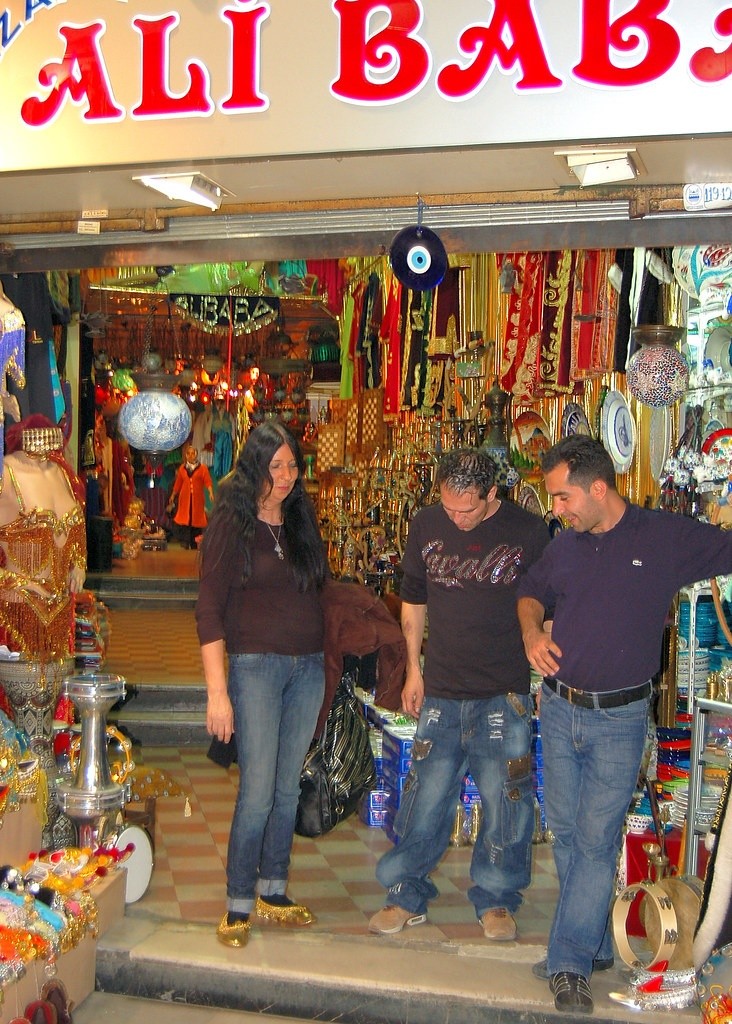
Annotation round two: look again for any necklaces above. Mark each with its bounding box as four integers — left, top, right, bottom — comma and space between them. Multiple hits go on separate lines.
264, 518, 284, 561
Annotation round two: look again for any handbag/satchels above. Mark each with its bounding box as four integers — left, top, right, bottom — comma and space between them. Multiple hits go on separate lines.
293, 655, 378, 839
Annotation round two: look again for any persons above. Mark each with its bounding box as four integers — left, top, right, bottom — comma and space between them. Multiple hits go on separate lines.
1, 429, 89, 695
170, 447, 213, 549
195, 425, 335, 949
369, 447, 557, 939
517, 435, 732, 1010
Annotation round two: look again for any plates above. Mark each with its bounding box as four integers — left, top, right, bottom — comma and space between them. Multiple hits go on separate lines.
508, 410, 552, 485
649, 405, 672, 481
517, 486, 544, 519
702, 428, 732, 468
561, 402, 591, 438
602, 390, 637, 474
544, 509, 564, 539
653, 713, 732, 830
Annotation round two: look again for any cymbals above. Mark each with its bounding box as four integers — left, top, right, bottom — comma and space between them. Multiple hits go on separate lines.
612, 877, 679, 971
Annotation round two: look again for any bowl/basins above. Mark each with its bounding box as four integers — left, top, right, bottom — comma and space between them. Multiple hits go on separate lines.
676, 603, 732, 697
625, 814, 653, 834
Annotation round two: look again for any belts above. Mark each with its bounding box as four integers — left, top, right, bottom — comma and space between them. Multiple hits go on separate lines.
543, 672, 652, 709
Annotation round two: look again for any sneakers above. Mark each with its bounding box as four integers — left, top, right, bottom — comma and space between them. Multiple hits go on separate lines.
549, 972, 594, 1014
532, 949, 614, 981
367, 903, 427, 934
478, 907, 518, 940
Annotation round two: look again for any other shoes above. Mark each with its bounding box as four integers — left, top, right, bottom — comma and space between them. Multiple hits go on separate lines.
216, 911, 252, 948
256, 895, 318, 926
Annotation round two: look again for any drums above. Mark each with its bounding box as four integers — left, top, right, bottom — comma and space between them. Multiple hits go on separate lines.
644, 875, 705, 971
